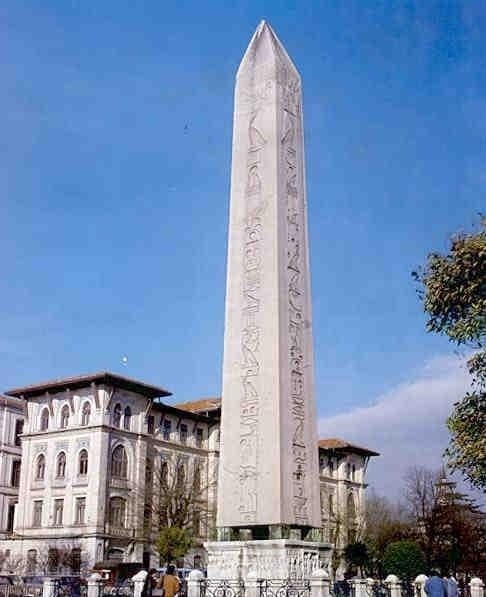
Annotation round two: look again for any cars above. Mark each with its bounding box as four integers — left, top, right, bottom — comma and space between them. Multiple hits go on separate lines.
0, 562, 207, 588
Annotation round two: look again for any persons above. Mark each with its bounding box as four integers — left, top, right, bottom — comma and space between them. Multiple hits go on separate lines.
139, 567, 158, 596
332, 570, 351, 597
157, 565, 182, 596
444, 569, 458, 597
453, 564, 466, 596
425, 568, 447, 596
467, 571, 485, 596
